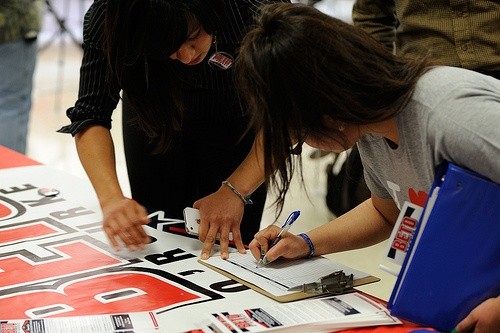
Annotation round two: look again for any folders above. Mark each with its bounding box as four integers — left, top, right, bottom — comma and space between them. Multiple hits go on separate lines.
387, 161, 500, 333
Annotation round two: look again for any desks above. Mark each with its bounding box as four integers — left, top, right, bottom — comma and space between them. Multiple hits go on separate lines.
1, 142, 436, 332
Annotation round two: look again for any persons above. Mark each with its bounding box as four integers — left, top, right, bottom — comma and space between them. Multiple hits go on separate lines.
352, 0, 499, 78
1, 0, 42, 158
235, 2, 500, 333
59, 0, 299, 260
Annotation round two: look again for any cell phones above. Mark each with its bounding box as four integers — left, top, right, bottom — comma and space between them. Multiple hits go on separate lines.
183, 207, 234, 241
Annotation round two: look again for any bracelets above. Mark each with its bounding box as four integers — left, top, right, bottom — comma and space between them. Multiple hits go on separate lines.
298, 233, 315, 259
222, 181, 253, 206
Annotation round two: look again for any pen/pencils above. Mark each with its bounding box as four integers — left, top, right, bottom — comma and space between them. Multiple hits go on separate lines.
255, 210, 301, 269
